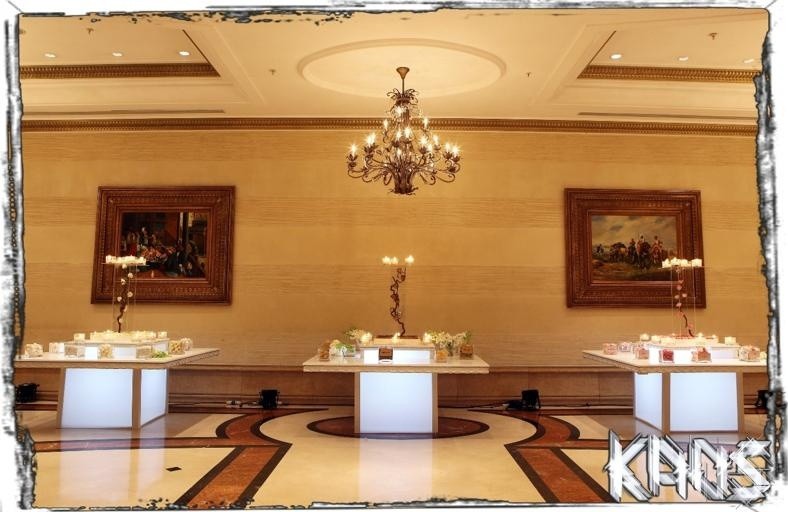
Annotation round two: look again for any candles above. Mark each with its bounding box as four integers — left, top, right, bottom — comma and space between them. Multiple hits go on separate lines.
640, 333, 649, 341
692, 258, 703, 266
670, 257, 680, 265
661, 336, 674, 345
405, 255, 414, 263
662, 258, 670, 267
725, 336, 736, 345
651, 335, 660, 344
382, 256, 392, 264
391, 257, 399, 263
681, 259, 688, 265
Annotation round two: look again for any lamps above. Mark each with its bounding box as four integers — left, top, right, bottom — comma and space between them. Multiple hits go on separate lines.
346, 66, 463, 196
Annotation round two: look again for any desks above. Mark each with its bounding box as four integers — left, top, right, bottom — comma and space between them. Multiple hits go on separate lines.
15, 331, 220, 429
302, 335, 490, 439
582, 333, 768, 442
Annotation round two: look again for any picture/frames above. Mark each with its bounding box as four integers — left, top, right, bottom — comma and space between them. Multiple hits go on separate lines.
91, 184, 236, 305
564, 187, 707, 309
